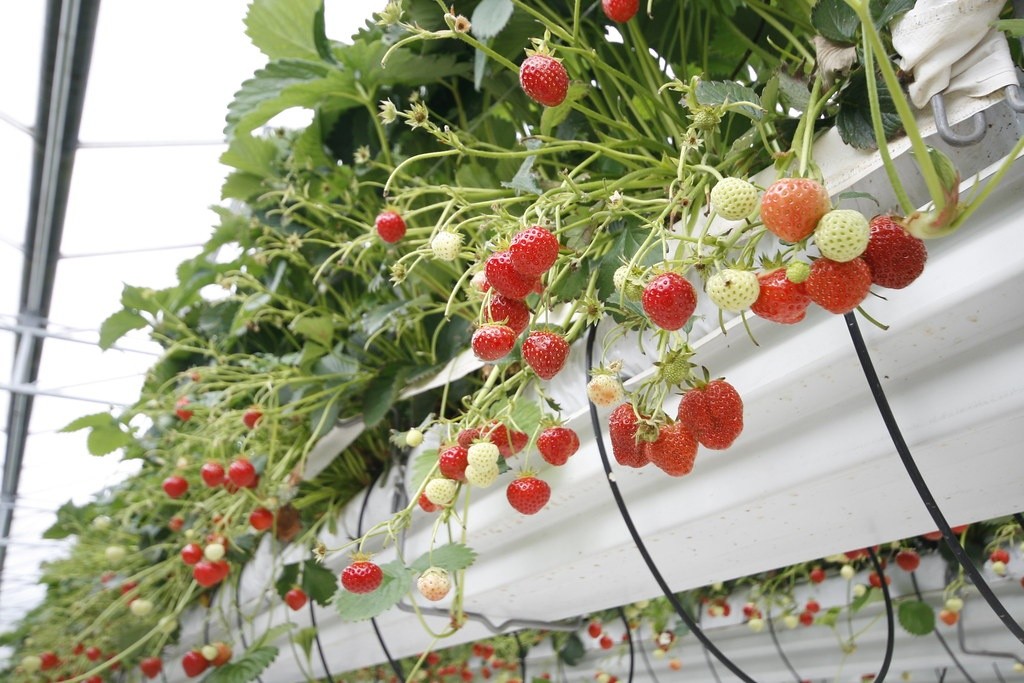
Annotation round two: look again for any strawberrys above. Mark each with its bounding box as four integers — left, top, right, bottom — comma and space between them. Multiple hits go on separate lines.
602, 0, 640, 24
374, 212, 407, 242
351, 521, 1024, 683
419, 177, 927, 514
518, 54, 567, 106
36, 366, 309, 681
342, 562, 384, 594
